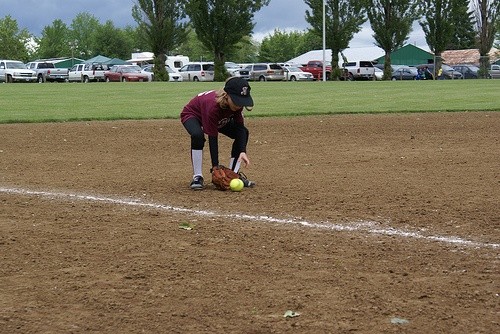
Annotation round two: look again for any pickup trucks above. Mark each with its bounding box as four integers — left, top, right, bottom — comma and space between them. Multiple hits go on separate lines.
24, 61, 69, 83
67, 63, 106, 83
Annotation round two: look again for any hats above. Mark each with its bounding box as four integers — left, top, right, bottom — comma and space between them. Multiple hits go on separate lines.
223, 77, 254, 107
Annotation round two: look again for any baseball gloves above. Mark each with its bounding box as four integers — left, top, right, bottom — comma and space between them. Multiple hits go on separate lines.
212, 166, 240, 191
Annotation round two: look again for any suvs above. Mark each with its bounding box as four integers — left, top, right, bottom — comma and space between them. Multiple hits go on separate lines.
339, 60, 375, 81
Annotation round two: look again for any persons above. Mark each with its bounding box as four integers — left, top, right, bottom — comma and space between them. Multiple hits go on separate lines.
180, 77, 254, 190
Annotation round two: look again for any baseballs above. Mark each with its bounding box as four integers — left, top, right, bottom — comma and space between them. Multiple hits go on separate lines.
230, 179, 244, 192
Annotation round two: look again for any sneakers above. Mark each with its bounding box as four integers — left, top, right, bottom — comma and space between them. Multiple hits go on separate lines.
236, 170, 255, 186
190, 174, 204, 188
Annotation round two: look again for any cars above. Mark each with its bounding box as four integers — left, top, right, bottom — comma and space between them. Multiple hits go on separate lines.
0, 59, 38, 84
373, 62, 500, 80
141, 63, 184, 82
103, 64, 152, 83
176, 61, 214, 82
223, 59, 333, 82
